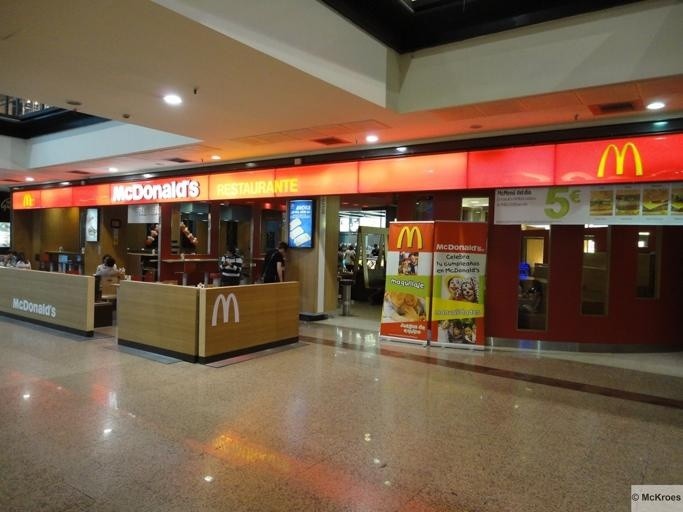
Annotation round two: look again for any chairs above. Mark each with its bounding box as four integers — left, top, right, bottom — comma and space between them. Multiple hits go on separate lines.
101, 277, 116, 302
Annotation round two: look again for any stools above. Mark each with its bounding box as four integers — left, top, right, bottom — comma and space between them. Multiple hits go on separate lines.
175, 269, 221, 286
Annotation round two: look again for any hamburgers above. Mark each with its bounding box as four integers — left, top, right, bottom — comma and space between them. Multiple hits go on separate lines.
615, 189, 638, 215
642, 191, 668, 211
671, 188, 683, 212
591, 190, 612, 215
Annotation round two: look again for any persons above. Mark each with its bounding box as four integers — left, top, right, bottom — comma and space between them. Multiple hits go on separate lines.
371, 244, 379, 256
262, 240, 287, 283
218, 246, 243, 286
0, 251, 32, 269
438, 319, 476, 344
338, 243, 356, 271
399, 253, 417, 274
95, 254, 117, 271
95, 258, 119, 276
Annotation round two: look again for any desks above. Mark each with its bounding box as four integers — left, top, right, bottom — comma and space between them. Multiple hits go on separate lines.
163, 258, 219, 287
